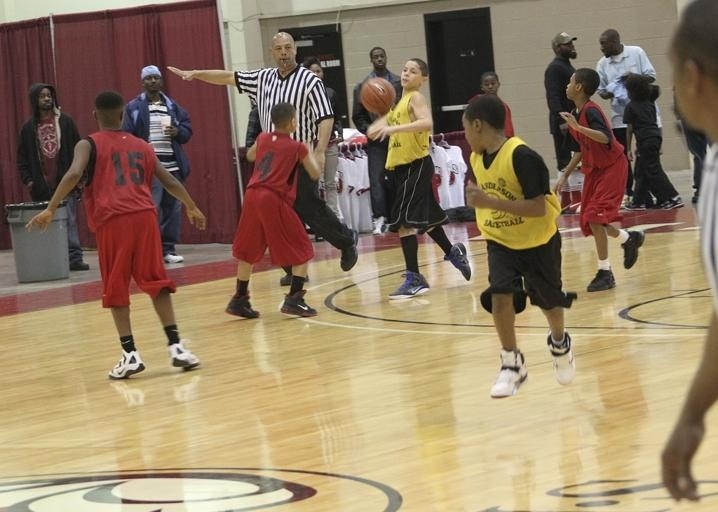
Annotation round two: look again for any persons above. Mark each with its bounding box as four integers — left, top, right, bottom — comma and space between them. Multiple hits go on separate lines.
15, 83, 90, 271
222, 28, 685, 318
673, 85, 710, 206
461, 94, 576, 398
120, 65, 194, 263
662, 0, 718, 502
24, 91, 207, 379
167, 32, 358, 285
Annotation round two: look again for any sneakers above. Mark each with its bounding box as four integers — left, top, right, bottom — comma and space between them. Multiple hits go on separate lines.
108, 348, 146, 380
339, 230, 358, 272
387, 269, 430, 301
560, 189, 698, 214
224, 291, 261, 319
446, 242, 471, 281
371, 217, 389, 237
166, 342, 201, 372
279, 288, 319, 317
546, 326, 576, 387
163, 253, 185, 264
279, 271, 309, 287
489, 347, 529, 398
620, 228, 646, 270
70, 258, 90, 271
587, 266, 616, 292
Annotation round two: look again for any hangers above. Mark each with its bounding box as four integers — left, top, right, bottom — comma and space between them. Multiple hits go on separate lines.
338, 132, 451, 159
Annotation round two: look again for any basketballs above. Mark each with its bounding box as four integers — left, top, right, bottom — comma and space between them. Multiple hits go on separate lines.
362, 79, 395, 113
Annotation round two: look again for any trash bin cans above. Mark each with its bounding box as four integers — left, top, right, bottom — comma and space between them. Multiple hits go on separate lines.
4, 199, 71, 283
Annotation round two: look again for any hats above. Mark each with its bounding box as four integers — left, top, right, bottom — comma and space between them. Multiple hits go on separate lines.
141, 65, 162, 81
551, 31, 578, 48
